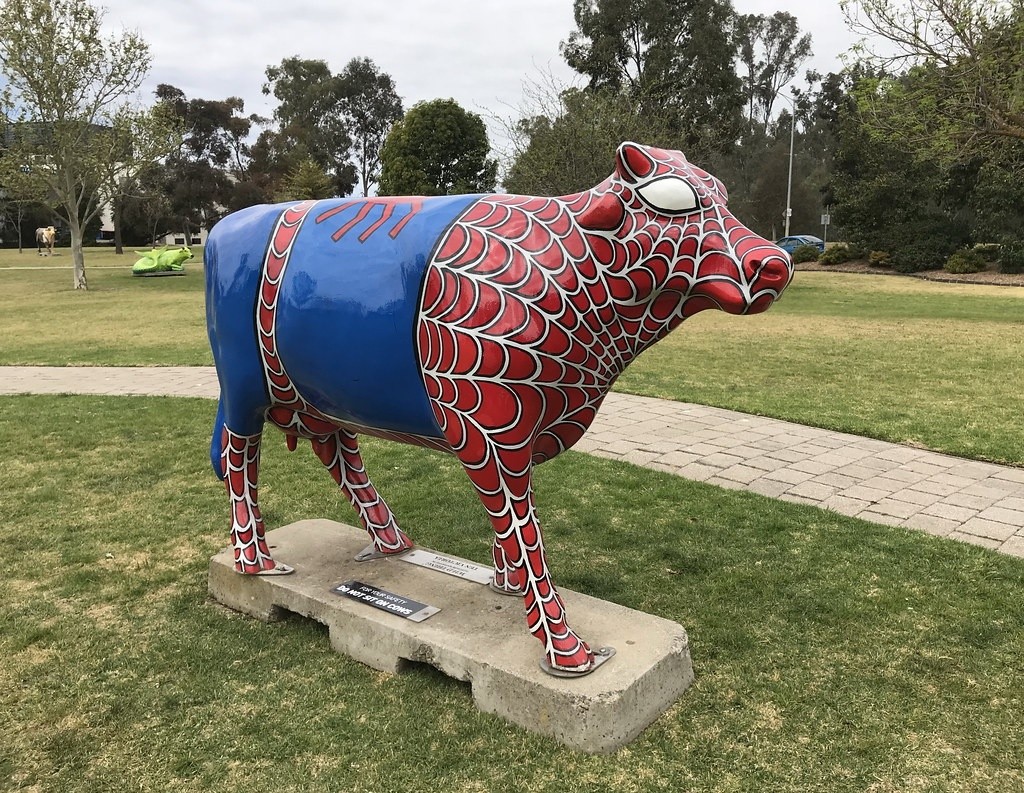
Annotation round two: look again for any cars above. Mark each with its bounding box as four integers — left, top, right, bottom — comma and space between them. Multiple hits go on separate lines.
775, 235, 824, 255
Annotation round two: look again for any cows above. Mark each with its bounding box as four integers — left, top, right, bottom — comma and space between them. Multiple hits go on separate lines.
36, 226, 57, 253
204, 140, 795, 674
